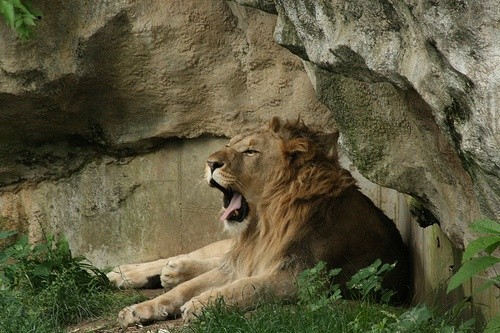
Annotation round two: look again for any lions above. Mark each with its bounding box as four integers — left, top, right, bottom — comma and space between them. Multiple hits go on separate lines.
107, 114, 416, 330
